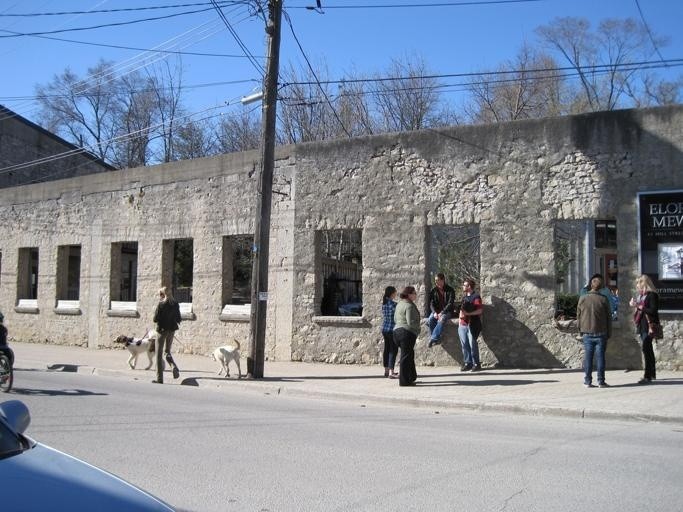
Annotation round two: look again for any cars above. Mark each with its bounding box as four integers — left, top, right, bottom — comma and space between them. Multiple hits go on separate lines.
0, 397, 178, 512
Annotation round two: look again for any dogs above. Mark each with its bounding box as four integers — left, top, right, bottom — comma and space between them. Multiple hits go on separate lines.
212, 338, 243, 379
112, 332, 156, 372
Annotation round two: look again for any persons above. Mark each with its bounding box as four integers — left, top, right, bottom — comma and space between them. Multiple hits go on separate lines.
393, 286, 421, 386
0, 312, 14, 384
629, 274, 660, 384
579, 273, 613, 313
380, 286, 398, 379
577, 278, 612, 388
427, 273, 455, 347
152, 287, 181, 384
458, 279, 484, 372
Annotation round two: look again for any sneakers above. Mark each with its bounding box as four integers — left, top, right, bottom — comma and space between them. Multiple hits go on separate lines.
461, 363, 472, 371
472, 363, 481, 371
173, 368, 179, 379
428, 339, 441, 348
599, 383, 607, 388
584, 383, 591, 387
152, 380, 163, 384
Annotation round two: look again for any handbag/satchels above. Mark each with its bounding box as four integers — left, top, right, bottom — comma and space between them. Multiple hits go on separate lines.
648, 323, 663, 339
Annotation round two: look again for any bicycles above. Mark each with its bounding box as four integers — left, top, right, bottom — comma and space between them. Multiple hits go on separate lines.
0, 351, 16, 395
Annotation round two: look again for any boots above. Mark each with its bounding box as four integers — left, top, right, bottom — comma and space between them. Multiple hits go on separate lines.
385, 367, 400, 379
